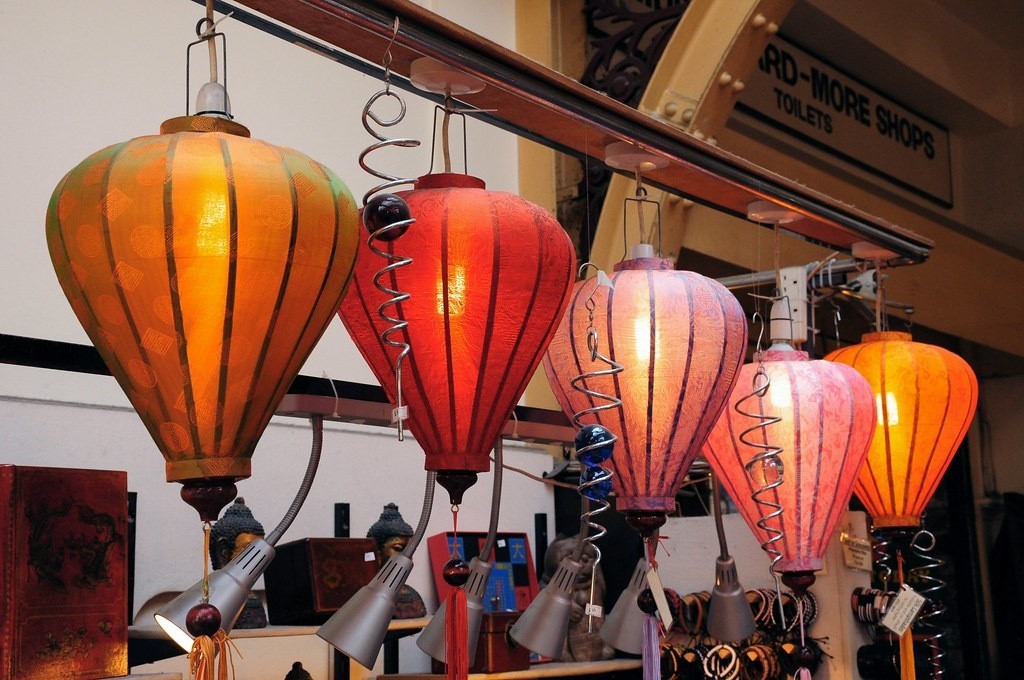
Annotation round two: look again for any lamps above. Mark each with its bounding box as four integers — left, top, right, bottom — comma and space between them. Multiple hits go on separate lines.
417, 441, 503, 664
506, 496, 589, 659
317, 472, 434, 667
151, 417, 323, 658
598, 532, 655, 655
706, 467, 756, 641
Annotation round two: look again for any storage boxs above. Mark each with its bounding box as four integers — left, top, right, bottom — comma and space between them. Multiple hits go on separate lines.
431, 610, 531, 673
427, 530, 556, 665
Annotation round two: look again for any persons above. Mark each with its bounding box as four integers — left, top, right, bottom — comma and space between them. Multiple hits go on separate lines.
366, 501, 428, 618
209, 497, 268, 629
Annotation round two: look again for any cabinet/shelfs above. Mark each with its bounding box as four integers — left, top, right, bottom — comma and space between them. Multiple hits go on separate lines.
226, 613, 642, 680
